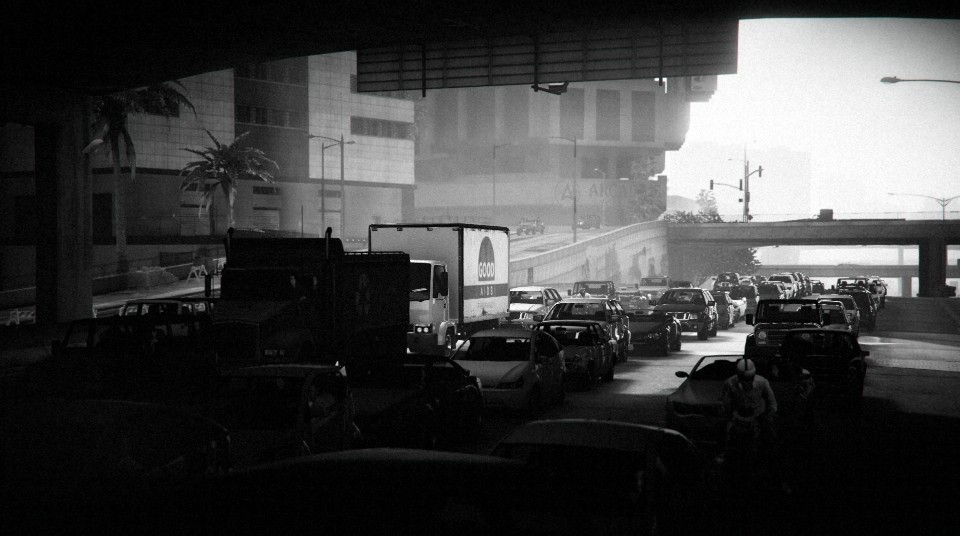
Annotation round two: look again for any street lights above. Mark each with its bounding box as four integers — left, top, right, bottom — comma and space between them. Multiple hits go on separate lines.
549, 135, 576, 243
492, 142, 518, 225
307, 134, 355, 241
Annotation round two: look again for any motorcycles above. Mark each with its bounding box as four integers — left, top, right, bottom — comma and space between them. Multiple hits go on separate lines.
720, 405, 768, 481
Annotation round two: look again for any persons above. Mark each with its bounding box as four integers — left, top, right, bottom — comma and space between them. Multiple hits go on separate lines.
577, 289, 590, 298
719, 358, 777, 434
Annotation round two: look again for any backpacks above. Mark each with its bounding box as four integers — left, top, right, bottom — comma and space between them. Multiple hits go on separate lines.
303, 372, 353, 452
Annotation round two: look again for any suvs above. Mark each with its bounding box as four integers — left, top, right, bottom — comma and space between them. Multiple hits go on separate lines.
0, 299, 210, 400
508, 287, 563, 332
517, 216, 545, 235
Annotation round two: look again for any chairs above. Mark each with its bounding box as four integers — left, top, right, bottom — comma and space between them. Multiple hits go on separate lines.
595, 311, 605, 320
800, 306, 815, 322
559, 311, 572, 319
766, 306, 779, 322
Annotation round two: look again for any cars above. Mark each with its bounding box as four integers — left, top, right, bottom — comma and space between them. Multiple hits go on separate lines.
200, 366, 351, 463
449, 329, 566, 420
714, 271, 887, 401
532, 323, 619, 386
346, 355, 484, 453
548, 271, 719, 358
667, 355, 740, 448
488, 418, 713, 536
213, 446, 566, 534
0, 398, 233, 536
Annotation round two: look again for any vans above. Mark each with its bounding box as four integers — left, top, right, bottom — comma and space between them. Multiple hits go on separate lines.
573, 214, 601, 230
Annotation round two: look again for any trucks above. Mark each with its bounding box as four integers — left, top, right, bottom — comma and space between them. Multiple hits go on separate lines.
369, 224, 512, 355
209, 228, 410, 367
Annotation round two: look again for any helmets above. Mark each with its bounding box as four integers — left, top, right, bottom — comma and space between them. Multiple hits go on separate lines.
737, 358, 755, 376
